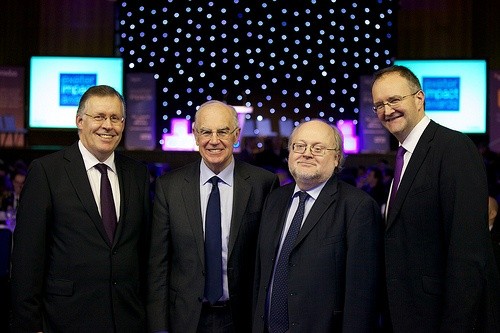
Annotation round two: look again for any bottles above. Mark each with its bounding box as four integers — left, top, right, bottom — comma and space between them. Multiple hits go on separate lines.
5, 206, 16, 229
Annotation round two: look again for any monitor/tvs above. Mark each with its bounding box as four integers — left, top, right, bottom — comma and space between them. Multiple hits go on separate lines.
390, 58, 490, 151
24, 54, 126, 131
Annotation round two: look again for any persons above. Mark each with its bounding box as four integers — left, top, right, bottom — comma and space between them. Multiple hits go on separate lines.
12, 86, 152, 333
488, 196, 499, 230
0, 169, 26, 211
147, 100, 281, 333
370, 66, 500, 333
250, 118, 386, 333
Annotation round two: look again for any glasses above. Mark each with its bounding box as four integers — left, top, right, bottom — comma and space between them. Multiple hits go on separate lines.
80, 112, 125, 123
195, 126, 239, 139
290, 143, 340, 156
372, 89, 420, 114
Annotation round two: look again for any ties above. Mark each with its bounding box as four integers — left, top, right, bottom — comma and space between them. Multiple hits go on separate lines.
93, 163, 117, 245
269, 192, 311, 333
203, 176, 223, 305
389, 145, 407, 206
16, 198, 19, 207
